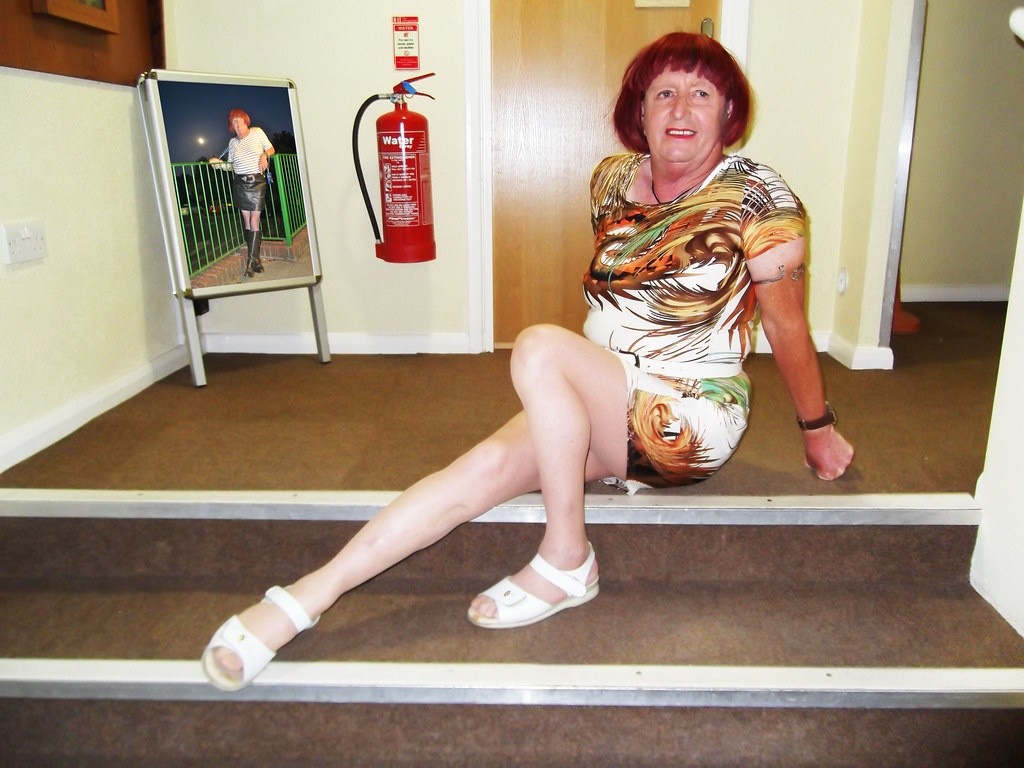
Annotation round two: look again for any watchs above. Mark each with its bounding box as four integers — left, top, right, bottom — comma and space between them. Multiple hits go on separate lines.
797, 401, 838, 432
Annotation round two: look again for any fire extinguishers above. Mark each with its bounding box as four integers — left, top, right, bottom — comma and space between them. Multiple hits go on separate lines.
352, 72, 437, 263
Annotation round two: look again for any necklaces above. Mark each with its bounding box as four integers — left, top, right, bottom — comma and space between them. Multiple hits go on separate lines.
651, 179, 690, 204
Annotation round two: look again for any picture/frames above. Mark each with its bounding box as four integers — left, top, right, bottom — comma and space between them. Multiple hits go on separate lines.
33, 0, 122, 34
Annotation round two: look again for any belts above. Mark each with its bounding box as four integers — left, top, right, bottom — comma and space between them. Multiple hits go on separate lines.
610, 349, 742, 380
235, 174, 260, 183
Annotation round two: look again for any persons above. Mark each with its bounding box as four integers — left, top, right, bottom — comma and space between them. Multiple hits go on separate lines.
210, 109, 274, 277
200, 30, 856, 692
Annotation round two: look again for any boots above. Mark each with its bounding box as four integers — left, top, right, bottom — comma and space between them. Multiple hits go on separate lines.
243, 229, 263, 272
245, 231, 261, 277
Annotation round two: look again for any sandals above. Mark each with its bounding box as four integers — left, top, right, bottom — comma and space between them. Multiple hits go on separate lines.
467, 541, 599, 629
202, 586, 321, 692
